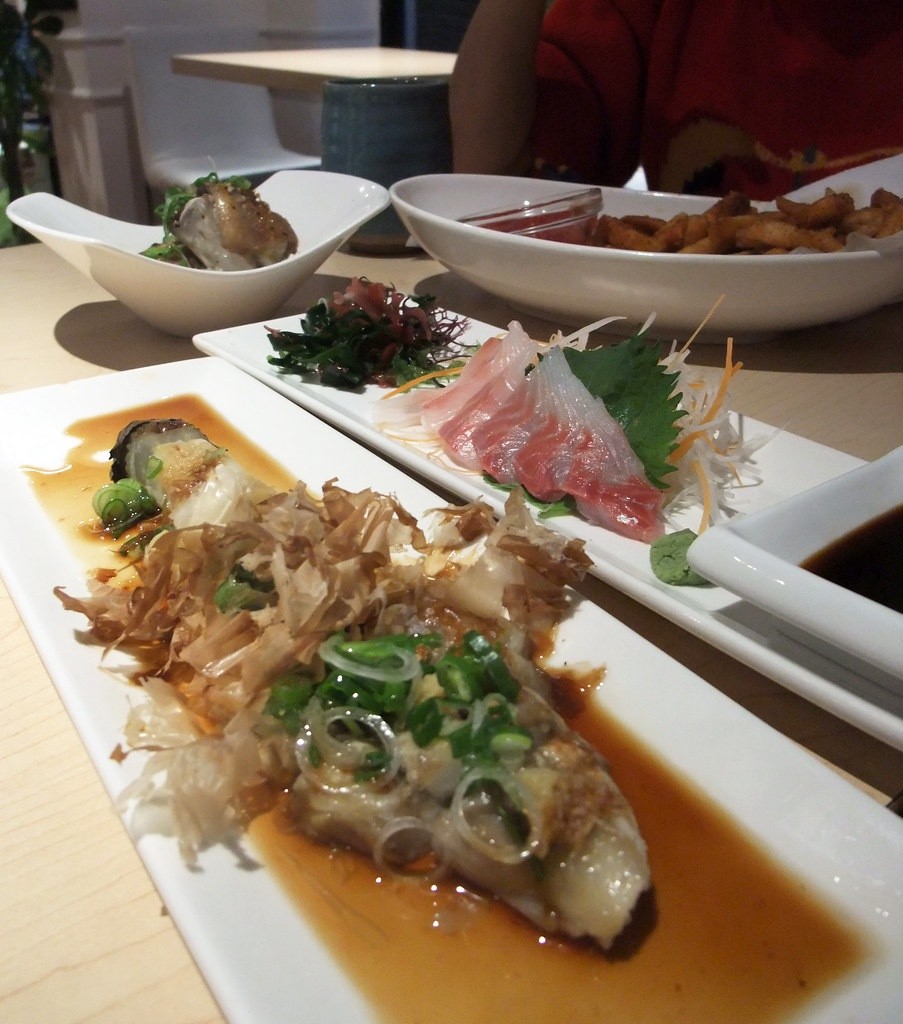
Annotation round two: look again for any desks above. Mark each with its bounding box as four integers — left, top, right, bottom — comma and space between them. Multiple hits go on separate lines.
175, 43, 470, 90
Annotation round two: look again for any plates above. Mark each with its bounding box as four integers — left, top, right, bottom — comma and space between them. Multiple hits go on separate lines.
0, 355, 903, 1024
192, 295, 903, 753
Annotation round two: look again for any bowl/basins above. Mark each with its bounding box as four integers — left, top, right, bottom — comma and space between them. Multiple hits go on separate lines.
684, 445, 903, 696
4, 168, 392, 339
390, 154, 903, 345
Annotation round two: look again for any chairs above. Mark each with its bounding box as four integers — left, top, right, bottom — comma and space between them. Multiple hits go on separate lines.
79, 1, 322, 191
38, 34, 151, 227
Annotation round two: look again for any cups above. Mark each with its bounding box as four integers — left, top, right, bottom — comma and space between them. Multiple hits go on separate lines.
321, 78, 454, 257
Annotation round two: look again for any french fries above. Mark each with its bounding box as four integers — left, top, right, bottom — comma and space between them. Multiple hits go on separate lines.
594, 188, 902, 253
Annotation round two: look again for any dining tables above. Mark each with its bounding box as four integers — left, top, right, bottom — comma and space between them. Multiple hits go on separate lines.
1, 233, 901, 1022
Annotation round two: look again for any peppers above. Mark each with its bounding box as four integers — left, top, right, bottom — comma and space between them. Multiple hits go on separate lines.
268, 626, 530, 790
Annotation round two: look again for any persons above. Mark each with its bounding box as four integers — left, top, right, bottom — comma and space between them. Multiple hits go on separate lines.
450, 0, 903, 205
